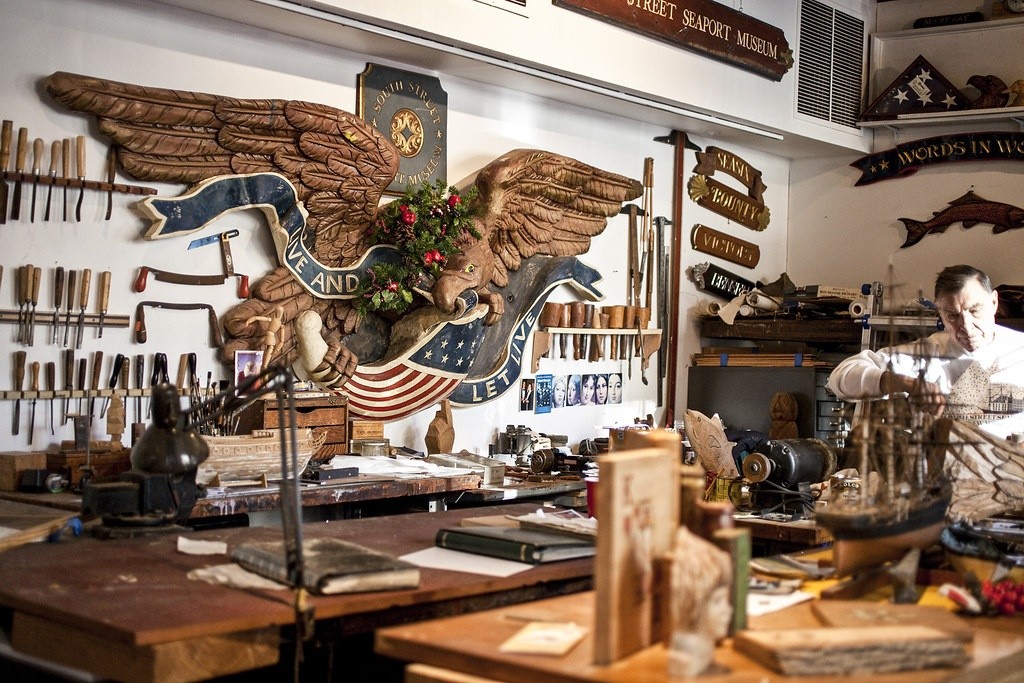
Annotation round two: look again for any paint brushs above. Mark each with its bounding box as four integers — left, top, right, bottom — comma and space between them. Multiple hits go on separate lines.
189, 365, 241, 436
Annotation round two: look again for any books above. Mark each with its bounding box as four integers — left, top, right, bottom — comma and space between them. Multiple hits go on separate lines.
229, 535, 425, 597
438, 524, 600, 565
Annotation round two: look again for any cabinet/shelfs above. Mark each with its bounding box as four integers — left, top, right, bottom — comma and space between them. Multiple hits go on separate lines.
855, 15, 1024, 143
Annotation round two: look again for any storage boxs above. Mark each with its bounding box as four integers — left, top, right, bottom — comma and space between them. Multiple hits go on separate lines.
688, 362, 862, 449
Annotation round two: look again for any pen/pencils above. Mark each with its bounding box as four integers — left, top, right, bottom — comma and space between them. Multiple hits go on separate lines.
779, 553, 811, 571
789, 556, 819, 564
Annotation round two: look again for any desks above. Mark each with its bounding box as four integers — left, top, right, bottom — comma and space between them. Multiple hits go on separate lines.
732, 509, 836, 560
0, 500, 597, 681
2, 475, 598, 556
374, 539, 1024, 681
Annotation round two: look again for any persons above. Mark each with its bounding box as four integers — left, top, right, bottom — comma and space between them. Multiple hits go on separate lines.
825, 265, 1024, 482
521, 372, 622, 410
